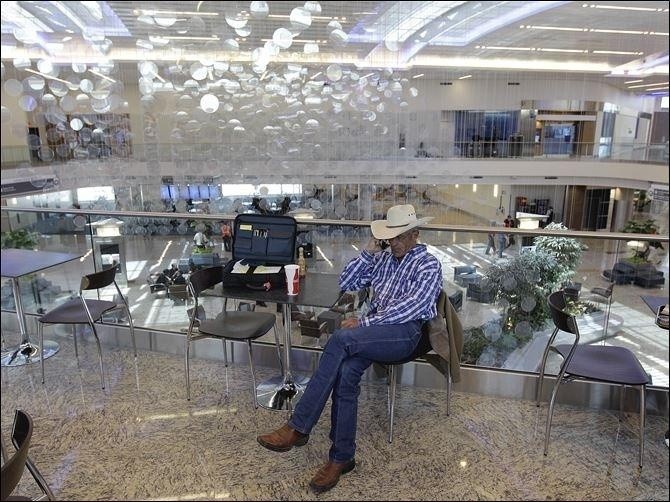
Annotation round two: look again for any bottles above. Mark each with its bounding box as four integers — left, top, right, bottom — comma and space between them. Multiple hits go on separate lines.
298, 247, 306, 278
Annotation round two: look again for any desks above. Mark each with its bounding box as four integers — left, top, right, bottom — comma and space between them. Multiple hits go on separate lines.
637, 295, 669, 449
187, 271, 347, 414
1, 247, 84, 368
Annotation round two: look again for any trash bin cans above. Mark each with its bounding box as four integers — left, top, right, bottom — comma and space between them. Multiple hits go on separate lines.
508, 135, 524, 158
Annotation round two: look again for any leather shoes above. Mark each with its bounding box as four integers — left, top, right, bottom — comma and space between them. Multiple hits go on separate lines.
310, 458, 355, 490
257, 424, 309, 452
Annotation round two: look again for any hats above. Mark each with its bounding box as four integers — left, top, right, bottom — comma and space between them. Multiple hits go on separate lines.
371, 204, 435, 239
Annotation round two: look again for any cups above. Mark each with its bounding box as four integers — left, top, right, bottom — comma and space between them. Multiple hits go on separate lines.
284, 263, 300, 296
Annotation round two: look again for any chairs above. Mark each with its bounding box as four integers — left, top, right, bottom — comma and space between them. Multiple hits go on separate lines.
379, 285, 452, 443
289, 291, 357, 348
535, 291, 652, 469
589, 279, 617, 307
182, 265, 283, 410
37, 259, 140, 391
144, 258, 192, 307
1, 407, 56, 502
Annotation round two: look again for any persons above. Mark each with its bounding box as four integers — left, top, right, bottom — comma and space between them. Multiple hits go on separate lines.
257, 202, 443, 490
543, 205, 554, 226
222, 223, 232, 250
486, 214, 518, 256
193, 229, 208, 249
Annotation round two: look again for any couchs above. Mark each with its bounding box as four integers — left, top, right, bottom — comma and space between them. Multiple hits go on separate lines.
451, 264, 484, 289
466, 283, 498, 304
602, 260, 666, 289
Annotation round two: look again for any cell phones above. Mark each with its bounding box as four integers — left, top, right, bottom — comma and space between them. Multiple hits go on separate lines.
379, 238, 390, 249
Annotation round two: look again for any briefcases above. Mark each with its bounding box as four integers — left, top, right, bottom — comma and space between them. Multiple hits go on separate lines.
222, 213, 297, 289
